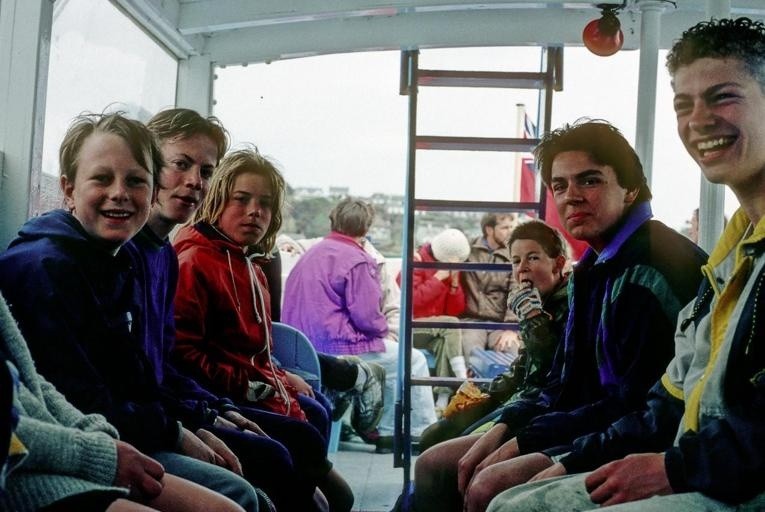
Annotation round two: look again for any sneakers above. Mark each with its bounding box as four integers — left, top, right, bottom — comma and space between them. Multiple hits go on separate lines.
376, 436, 394, 453
340, 420, 357, 442
351, 356, 387, 434
325, 390, 354, 422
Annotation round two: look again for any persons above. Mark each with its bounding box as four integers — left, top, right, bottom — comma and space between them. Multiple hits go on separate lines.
0, 108, 263, 512
458, 212, 524, 357
417, 219, 574, 456
481, 17, 765, 511
280, 197, 439, 437
167, 142, 334, 454
415, 113, 712, 510
396, 226, 473, 416
249, 238, 387, 436
0, 294, 250, 511
128, 109, 328, 511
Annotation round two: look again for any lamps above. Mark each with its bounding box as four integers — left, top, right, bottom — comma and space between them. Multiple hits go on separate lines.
583, 0, 627, 56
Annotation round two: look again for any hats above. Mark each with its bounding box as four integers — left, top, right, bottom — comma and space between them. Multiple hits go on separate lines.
431, 229, 471, 264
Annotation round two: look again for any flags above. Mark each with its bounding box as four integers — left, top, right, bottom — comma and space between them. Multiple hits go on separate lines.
514, 103, 591, 261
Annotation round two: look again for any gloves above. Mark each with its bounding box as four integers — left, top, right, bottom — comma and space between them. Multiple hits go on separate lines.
507, 280, 543, 321
